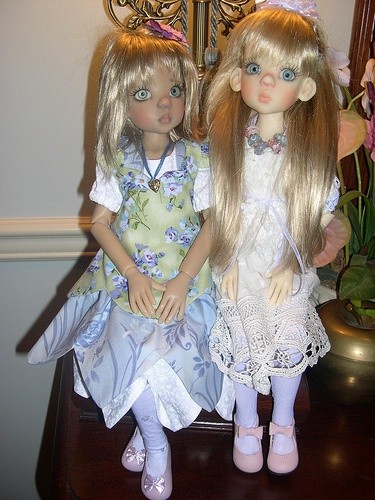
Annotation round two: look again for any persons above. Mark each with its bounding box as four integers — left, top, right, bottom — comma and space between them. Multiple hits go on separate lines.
202, 0, 341, 476
27, 18, 224, 500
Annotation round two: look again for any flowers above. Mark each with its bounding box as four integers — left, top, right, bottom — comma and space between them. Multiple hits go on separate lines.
313, 47, 375, 317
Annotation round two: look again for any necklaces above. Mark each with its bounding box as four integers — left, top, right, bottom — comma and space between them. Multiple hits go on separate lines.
139, 137, 173, 193
244, 114, 287, 156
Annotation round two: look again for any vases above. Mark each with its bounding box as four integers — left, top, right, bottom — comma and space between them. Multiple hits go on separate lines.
313, 248, 375, 305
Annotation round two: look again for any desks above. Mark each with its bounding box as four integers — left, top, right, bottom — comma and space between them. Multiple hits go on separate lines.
33, 349, 374, 500
